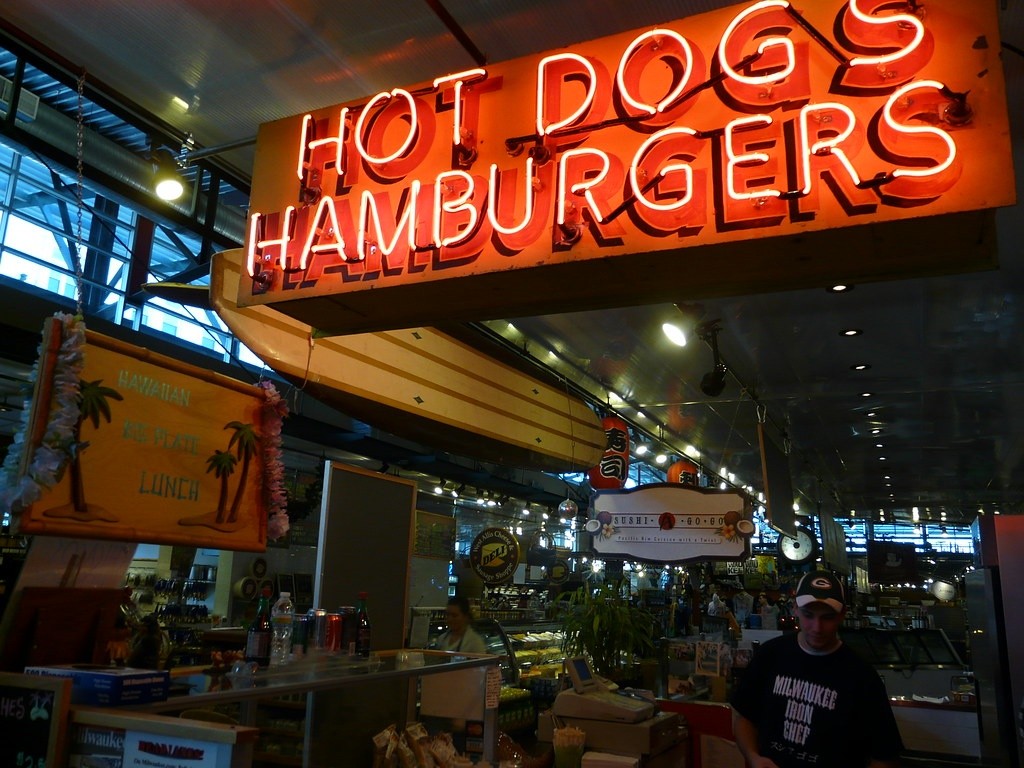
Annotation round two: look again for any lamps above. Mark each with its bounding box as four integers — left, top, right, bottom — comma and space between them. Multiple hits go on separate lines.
451, 485, 466, 498
434, 479, 446, 495
153, 149, 183, 201
475, 489, 484, 503
493, 493, 511, 507
661, 300, 709, 348
653, 440, 666, 464
633, 430, 648, 455
701, 320, 729, 397
487, 490, 497, 506
378, 465, 389, 473
523, 502, 531, 515
940, 527, 948, 534
543, 506, 553, 518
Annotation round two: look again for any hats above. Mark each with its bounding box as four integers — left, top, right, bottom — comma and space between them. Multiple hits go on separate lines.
794, 570, 845, 613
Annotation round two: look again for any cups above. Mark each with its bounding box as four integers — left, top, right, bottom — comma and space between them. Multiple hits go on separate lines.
552, 733, 585, 768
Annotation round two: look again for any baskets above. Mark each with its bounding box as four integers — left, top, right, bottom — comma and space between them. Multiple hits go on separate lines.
27, 664, 171, 709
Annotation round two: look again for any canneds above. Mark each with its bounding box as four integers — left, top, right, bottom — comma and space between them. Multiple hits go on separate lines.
291, 606, 357, 656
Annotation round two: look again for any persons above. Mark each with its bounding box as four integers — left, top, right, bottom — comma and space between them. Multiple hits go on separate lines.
433, 597, 486, 654
731, 570, 907, 768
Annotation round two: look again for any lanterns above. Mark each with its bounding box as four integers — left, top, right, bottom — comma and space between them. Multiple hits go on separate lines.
590, 416, 630, 491
667, 461, 698, 485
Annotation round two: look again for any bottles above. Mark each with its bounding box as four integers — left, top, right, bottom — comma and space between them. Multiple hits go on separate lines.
272, 592, 294, 665
244, 588, 272, 669
499, 673, 571, 723
911, 610, 929, 629
354, 592, 371, 655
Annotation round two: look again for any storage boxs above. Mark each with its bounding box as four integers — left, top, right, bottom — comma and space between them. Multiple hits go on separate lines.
25, 662, 172, 709
